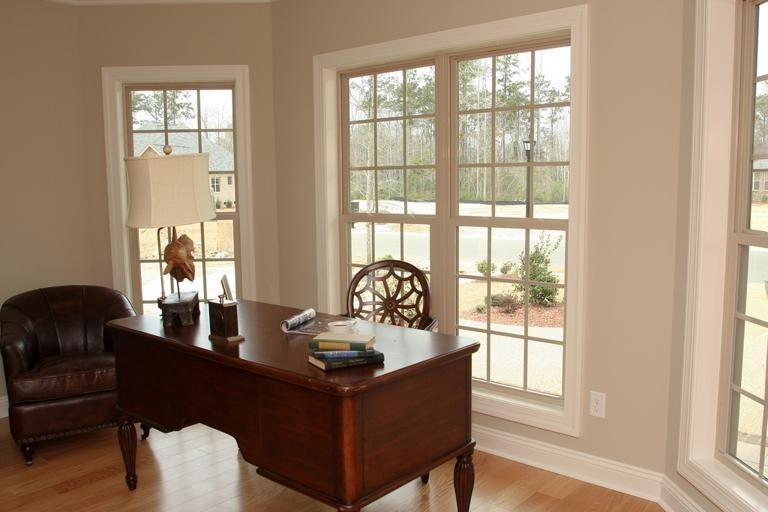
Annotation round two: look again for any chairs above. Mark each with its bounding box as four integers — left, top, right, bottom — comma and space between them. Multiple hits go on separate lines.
0, 285, 150, 465
334, 260, 438, 484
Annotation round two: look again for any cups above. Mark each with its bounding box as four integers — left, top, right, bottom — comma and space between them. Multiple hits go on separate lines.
328, 320, 355, 335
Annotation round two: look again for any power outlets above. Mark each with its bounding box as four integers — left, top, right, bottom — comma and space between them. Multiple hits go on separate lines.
590, 390, 605, 418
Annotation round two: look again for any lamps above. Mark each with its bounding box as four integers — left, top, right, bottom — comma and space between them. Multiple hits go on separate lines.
124, 145, 217, 328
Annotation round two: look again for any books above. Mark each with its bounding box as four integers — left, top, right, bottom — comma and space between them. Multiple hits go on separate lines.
311, 348, 377, 359
308, 332, 377, 352
281, 307, 358, 336
307, 349, 384, 372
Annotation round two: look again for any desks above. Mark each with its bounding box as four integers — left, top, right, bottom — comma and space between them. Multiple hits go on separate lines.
105, 298, 481, 512
